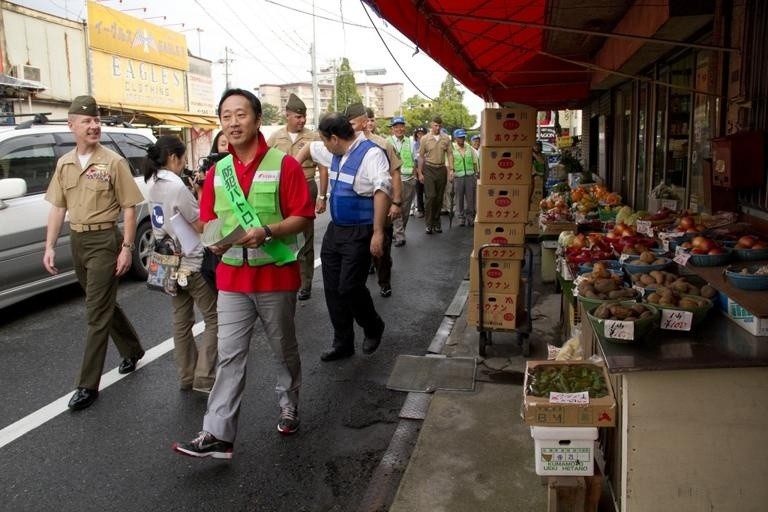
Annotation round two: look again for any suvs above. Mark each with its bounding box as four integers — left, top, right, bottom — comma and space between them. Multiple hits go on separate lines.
0, 119, 166, 311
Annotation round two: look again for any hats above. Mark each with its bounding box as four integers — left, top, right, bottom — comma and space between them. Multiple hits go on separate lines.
342, 103, 367, 122
391, 116, 482, 141
68, 96, 101, 116
286, 93, 306, 114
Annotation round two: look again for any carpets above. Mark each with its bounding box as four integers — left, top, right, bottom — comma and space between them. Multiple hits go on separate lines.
383, 354, 478, 392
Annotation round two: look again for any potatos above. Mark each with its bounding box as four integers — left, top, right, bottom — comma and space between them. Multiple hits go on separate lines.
571, 263, 654, 300
627, 271, 716, 311
590, 298, 658, 324
622, 244, 670, 270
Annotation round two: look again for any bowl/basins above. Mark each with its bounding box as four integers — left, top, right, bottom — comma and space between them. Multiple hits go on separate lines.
565, 213, 768, 344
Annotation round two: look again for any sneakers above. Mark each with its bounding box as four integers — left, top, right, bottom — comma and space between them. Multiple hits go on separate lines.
278, 406, 300, 434
173, 430, 234, 460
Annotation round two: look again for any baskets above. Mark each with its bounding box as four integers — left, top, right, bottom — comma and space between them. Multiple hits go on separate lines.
569, 224, 768, 345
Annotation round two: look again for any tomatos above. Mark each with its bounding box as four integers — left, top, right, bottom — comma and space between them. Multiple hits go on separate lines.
677, 236, 722, 257
675, 216, 705, 234
732, 234, 763, 251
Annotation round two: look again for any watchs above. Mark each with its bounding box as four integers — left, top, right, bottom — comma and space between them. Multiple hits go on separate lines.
262, 225, 273, 243
121, 241, 136, 252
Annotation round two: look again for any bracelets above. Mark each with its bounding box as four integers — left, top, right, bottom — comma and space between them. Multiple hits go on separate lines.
319, 195, 328, 201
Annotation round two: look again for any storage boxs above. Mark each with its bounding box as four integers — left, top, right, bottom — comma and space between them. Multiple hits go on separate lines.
479, 107, 537, 148
538, 219, 579, 236
471, 250, 523, 296
474, 216, 524, 258
713, 289, 768, 338
530, 173, 542, 209
531, 427, 596, 476
525, 211, 542, 234
647, 194, 682, 217
520, 359, 615, 428
468, 293, 518, 331
477, 184, 530, 223
478, 149, 533, 186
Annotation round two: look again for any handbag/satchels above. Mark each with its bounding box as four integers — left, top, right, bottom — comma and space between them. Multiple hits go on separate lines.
147, 251, 180, 297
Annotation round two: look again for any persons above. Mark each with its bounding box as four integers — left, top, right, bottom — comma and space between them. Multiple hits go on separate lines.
172, 86, 316, 461
408, 117, 481, 233
42, 96, 144, 410
384, 117, 416, 246
266, 93, 329, 300
143, 135, 219, 393
294, 112, 392, 361
535, 141, 548, 185
210, 130, 230, 154
346, 103, 404, 296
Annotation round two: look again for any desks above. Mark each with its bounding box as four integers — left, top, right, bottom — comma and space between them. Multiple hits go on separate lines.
539, 234, 559, 284
559, 238, 768, 511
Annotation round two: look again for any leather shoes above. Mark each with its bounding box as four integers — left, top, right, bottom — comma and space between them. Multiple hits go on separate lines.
68, 388, 98, 409
299, 286, 392, 361
395, 224, 442, 247
119, 350, 144, 373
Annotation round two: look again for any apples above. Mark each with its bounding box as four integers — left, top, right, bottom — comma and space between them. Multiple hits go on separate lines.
604, 221, 660, 251
556, 227, 616, 263
